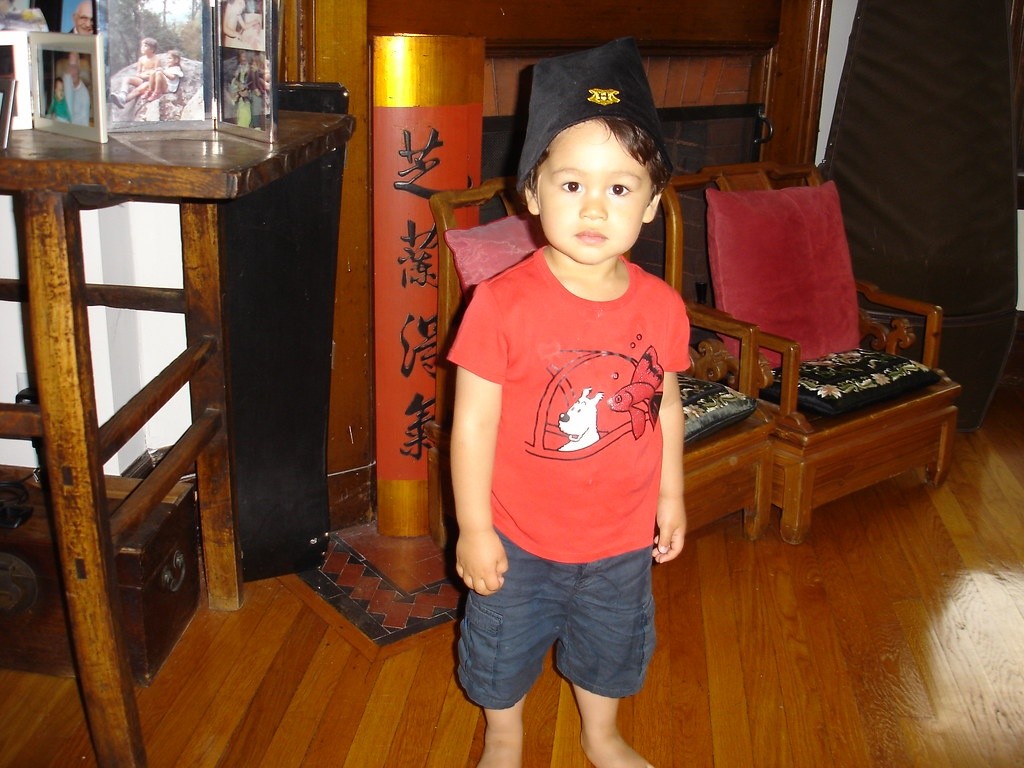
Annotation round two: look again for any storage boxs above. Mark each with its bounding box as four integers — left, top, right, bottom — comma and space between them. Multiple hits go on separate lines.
0, 462, 201, 685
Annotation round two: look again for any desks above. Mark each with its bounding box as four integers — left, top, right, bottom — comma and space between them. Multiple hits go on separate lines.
0, 112, 358, 768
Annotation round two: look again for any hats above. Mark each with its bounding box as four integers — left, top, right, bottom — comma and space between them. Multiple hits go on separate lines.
519, 36, 673, 192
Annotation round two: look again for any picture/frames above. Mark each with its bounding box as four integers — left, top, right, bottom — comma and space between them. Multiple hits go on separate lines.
0, 78, 16, 150
208, 0, 278, 144
29, 30, 108, 143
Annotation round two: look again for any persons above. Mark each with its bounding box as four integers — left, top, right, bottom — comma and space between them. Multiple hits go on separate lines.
68, 1, 94, 35
222, 1, 264, 51
141, 50, 183, 101
442, 39, 690, 768
46, 52, 91, 127
230, 50, 271, 131
110, 38, 162, 109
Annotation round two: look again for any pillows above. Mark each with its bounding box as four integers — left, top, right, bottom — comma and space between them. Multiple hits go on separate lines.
676, 370, 759, 447
706, 181, 943, 416
444, 210, 550, 315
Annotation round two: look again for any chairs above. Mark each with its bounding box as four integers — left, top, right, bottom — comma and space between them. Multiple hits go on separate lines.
429, 161, 962, 546
